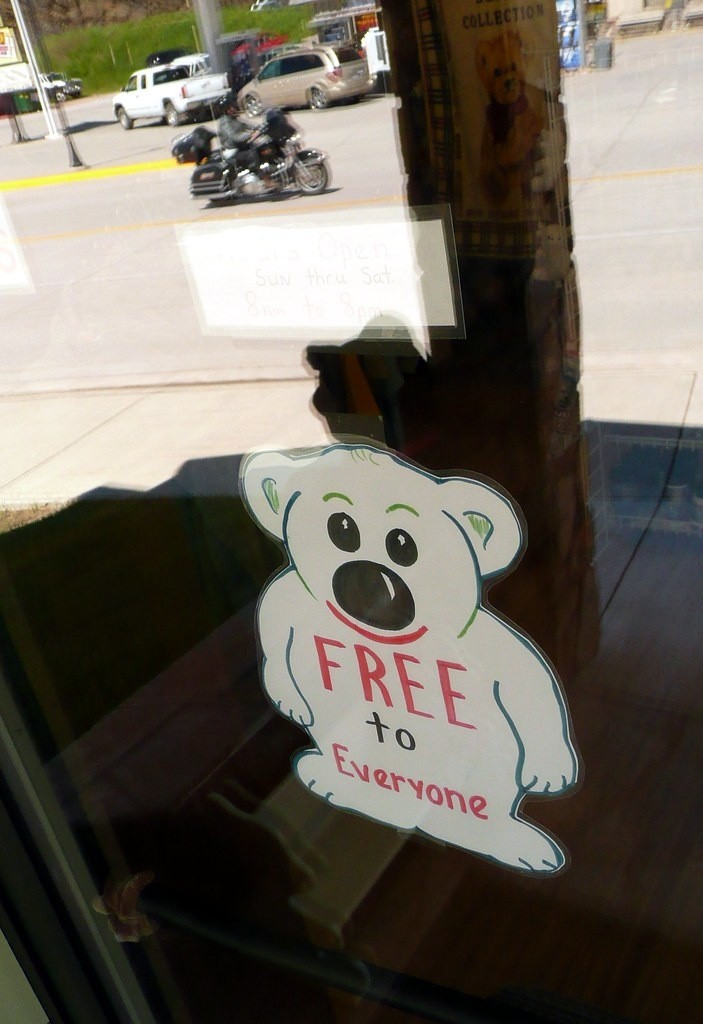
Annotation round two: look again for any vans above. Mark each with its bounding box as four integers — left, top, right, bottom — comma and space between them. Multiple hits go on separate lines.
173, 51, 211, 75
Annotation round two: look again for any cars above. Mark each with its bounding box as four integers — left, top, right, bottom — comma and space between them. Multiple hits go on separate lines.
219, 28, 288, 87
40, 70, 84, 102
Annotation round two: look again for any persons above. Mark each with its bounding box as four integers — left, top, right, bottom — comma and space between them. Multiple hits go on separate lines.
219, 97, 270, 175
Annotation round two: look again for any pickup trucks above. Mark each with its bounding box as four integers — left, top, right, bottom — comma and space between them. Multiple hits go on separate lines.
112, 65, 232, 131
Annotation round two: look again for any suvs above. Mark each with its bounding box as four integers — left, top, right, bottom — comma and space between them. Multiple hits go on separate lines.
236, 45, 370, 120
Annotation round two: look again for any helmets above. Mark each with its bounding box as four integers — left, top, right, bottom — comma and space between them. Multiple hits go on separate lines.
215, 96, 235, 115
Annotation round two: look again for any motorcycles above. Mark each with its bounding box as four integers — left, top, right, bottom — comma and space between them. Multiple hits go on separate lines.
171, 106, 329, 207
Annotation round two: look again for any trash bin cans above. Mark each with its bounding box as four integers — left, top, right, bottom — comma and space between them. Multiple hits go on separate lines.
593, 38, 613, 69
